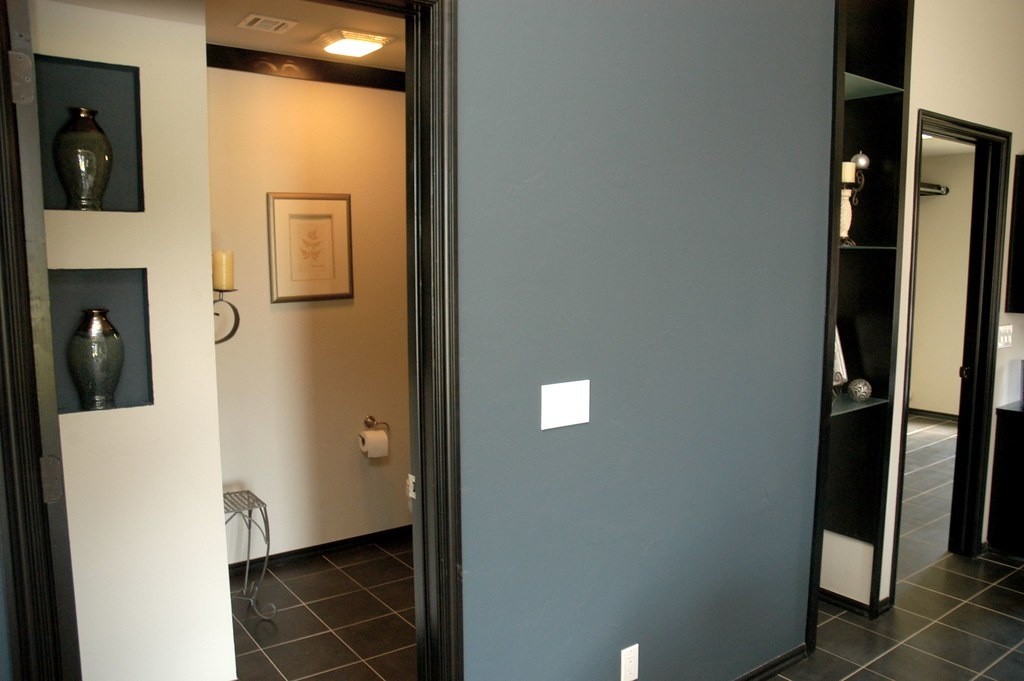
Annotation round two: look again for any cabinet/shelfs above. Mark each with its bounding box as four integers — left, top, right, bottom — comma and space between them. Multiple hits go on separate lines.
824, 0, 918, 418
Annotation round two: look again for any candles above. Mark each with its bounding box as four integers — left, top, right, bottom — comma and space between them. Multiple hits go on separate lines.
212, 249, 235, 291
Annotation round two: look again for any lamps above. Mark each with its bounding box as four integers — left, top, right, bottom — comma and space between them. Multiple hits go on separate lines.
312, 27, 399, 57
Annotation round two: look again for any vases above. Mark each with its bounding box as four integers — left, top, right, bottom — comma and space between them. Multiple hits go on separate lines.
66, 309, 125, 411
52, 107, 115, 211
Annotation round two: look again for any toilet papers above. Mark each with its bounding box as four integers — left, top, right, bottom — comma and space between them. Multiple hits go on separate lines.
358, 430, 388, 458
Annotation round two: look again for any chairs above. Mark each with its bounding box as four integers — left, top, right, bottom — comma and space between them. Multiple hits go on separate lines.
224, 490, 278, 620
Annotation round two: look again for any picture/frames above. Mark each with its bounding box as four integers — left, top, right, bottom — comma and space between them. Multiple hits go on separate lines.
266, 191, 355, 304
